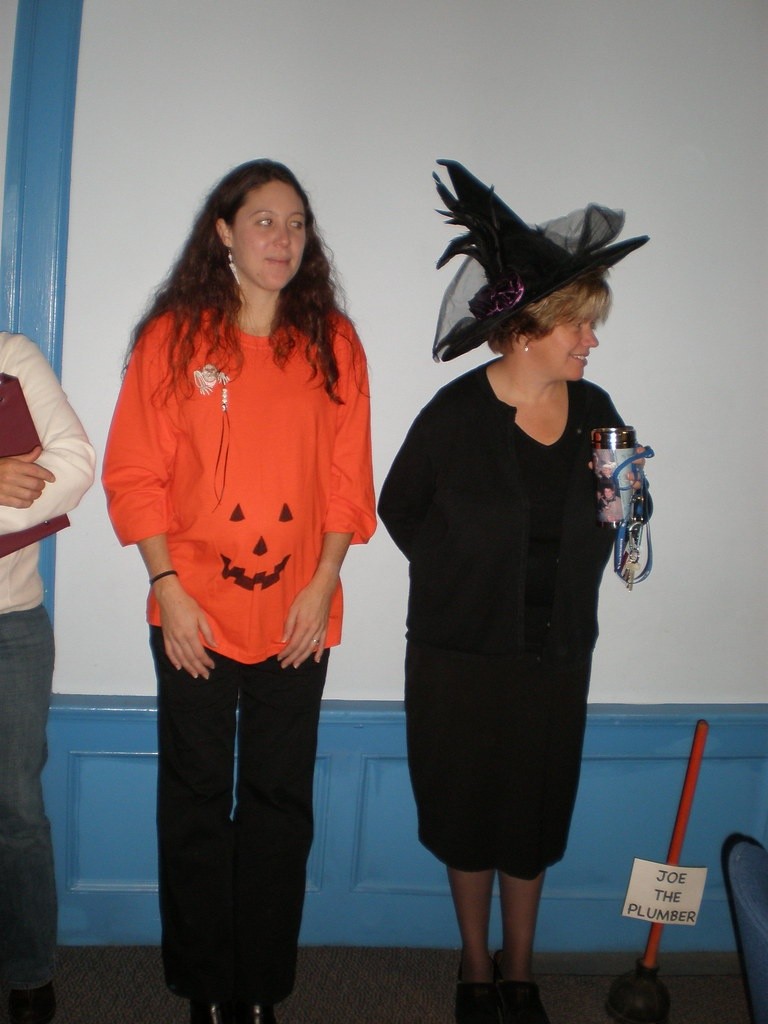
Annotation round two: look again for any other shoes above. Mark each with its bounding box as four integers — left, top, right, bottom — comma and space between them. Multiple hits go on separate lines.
492, 950, 549, 1024
454, 956, 502, 1024
8, 980, 57, 1024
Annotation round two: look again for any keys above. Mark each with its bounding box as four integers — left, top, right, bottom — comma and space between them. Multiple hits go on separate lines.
625, 560, 641, 592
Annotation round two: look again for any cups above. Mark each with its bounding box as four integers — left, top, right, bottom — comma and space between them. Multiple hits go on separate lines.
592, 427, 635, 522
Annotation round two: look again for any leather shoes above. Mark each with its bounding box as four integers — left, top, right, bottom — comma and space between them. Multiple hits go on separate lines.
186, 998, 277, 1024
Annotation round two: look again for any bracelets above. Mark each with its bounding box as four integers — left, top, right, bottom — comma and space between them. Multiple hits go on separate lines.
150, 570, 178, 586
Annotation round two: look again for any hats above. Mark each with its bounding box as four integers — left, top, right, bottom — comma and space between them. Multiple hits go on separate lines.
430, 157, 655, 364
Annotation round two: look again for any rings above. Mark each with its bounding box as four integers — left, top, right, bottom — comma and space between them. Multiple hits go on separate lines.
313, 639, 320, 644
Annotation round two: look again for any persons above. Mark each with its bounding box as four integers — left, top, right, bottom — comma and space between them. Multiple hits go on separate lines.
102, 158, 377, 1024
594, 455, 623, 522
0, 332, 96, 1024
377, 158, 654, 1024
